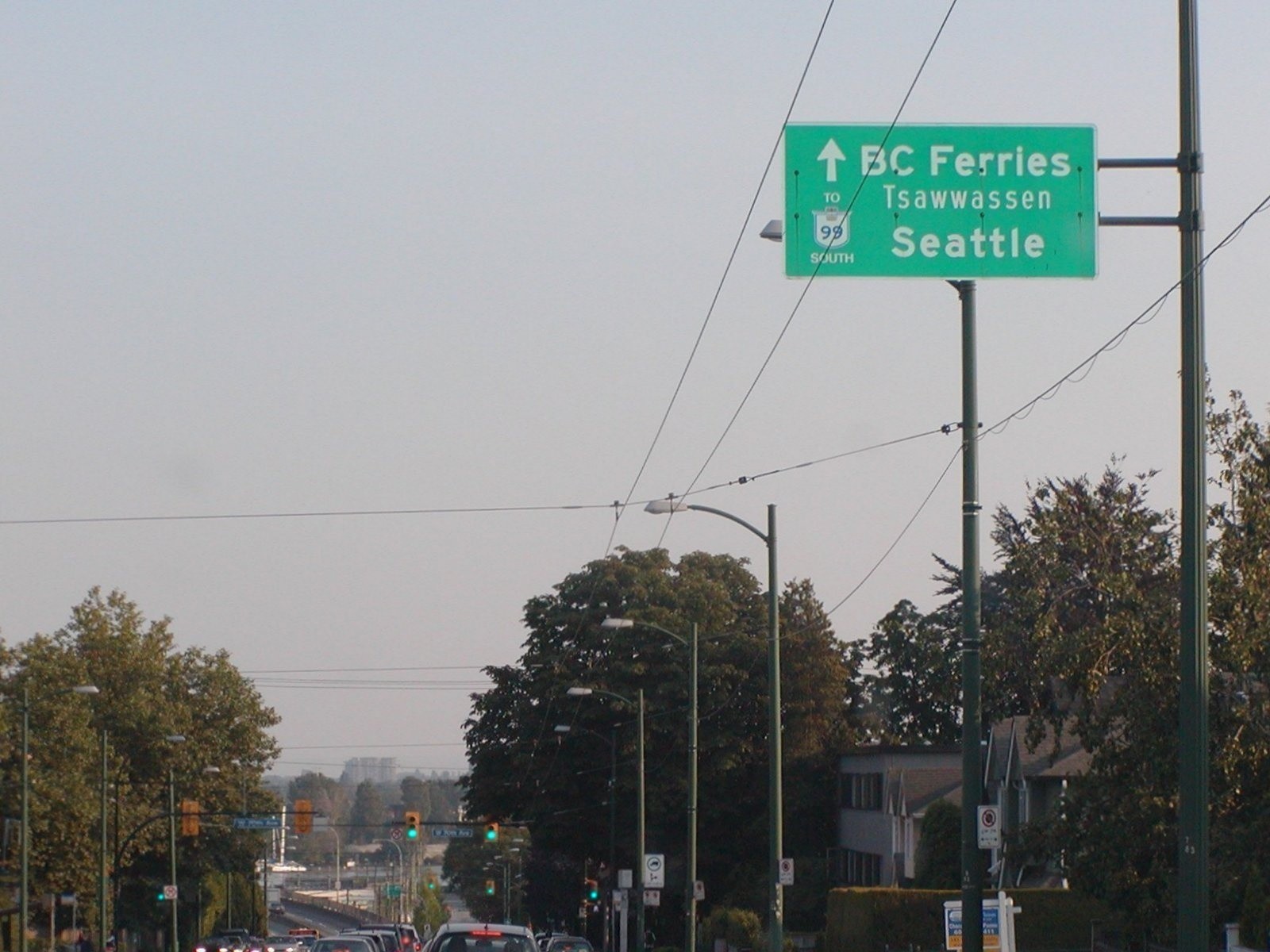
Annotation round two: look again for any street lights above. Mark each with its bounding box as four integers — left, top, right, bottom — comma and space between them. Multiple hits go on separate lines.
244, 824, 301, 937
20, 685, 219, 951
482, 847, 525, 923
552, 725, 618, 952
566, 687, 648, 952
230, 758, 249, 818
760, 217, 989, 952
371, 836, 405, 923
645, 498, 786, 952
598, 618, 698, 952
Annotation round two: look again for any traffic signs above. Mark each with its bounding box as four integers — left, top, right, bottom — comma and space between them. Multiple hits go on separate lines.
784, 120, 1097, 276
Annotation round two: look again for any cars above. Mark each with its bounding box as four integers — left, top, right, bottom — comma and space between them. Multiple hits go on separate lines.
193, 920, 422, 952
425, 921, 594, 952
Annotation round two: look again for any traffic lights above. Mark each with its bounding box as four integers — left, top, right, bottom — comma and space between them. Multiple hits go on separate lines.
404, 811, 421, 842
484, 822, 499, 844
485, 880, 496, 896
587, 879, 599, 901
